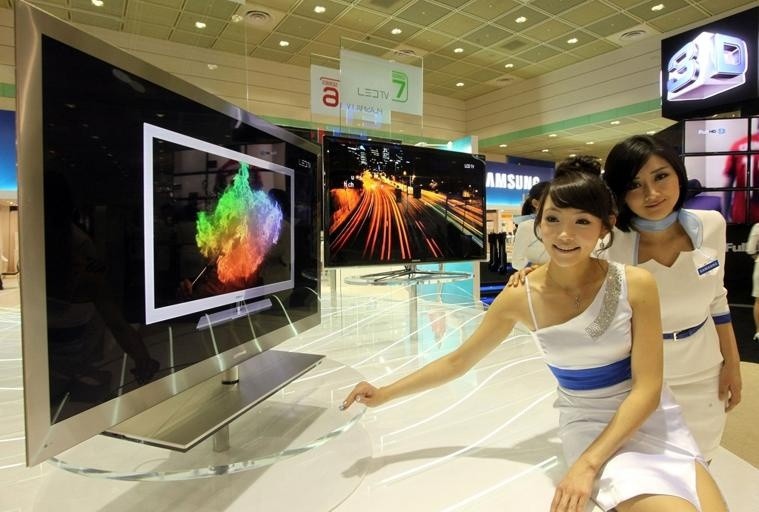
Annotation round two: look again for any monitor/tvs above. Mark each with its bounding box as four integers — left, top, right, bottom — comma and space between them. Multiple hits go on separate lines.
321, 134, 489, 283
140, 121, 296, 332
661, 2, 759, 120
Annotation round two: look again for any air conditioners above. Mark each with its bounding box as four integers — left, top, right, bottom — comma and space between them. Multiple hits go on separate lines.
236, 2, 286, 30
382, 44, 428, 63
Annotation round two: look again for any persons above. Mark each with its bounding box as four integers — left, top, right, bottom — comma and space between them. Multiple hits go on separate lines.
746, 224, 759, 337
511, 182, 554, 267
341, 155, 731, 512
507, 135, 742, 466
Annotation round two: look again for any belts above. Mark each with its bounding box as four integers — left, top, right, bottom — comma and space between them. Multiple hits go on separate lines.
662, 317, 710, 342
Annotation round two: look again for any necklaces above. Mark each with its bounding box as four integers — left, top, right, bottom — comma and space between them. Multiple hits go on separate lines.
548, 272, 602, 310
